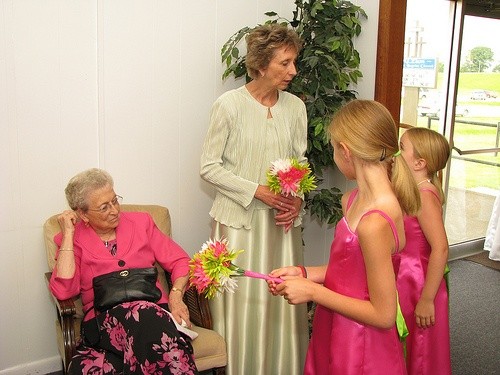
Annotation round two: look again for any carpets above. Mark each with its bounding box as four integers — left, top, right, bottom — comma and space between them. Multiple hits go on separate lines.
464, 251, 500, 271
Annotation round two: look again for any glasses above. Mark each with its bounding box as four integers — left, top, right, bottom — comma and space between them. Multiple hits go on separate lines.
85, 194, 123, 212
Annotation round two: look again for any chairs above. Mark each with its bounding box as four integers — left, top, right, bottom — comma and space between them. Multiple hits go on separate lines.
44, 205, 227, 375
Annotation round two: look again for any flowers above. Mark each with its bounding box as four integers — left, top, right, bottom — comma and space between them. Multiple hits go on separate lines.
186, 235, 284, 300
265, 156, 319, 202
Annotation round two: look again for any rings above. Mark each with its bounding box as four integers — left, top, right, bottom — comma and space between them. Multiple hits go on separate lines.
280, 203, 284, 206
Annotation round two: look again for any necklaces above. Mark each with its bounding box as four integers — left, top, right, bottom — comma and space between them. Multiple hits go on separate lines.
100, 227, 116, 248
417, 179, 427, 186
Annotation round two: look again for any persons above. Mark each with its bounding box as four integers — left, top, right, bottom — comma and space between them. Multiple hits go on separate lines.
49, 168, 200, 375
265, 101, 450, 375
201, 29, 307, 375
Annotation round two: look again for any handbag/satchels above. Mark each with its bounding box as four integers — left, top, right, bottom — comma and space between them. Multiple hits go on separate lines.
92, 266, 162, 313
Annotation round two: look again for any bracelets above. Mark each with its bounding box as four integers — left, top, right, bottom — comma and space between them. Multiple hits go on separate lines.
296, 265, 307, 278
59, 248, 75, 251
171, 288, 185, 294
